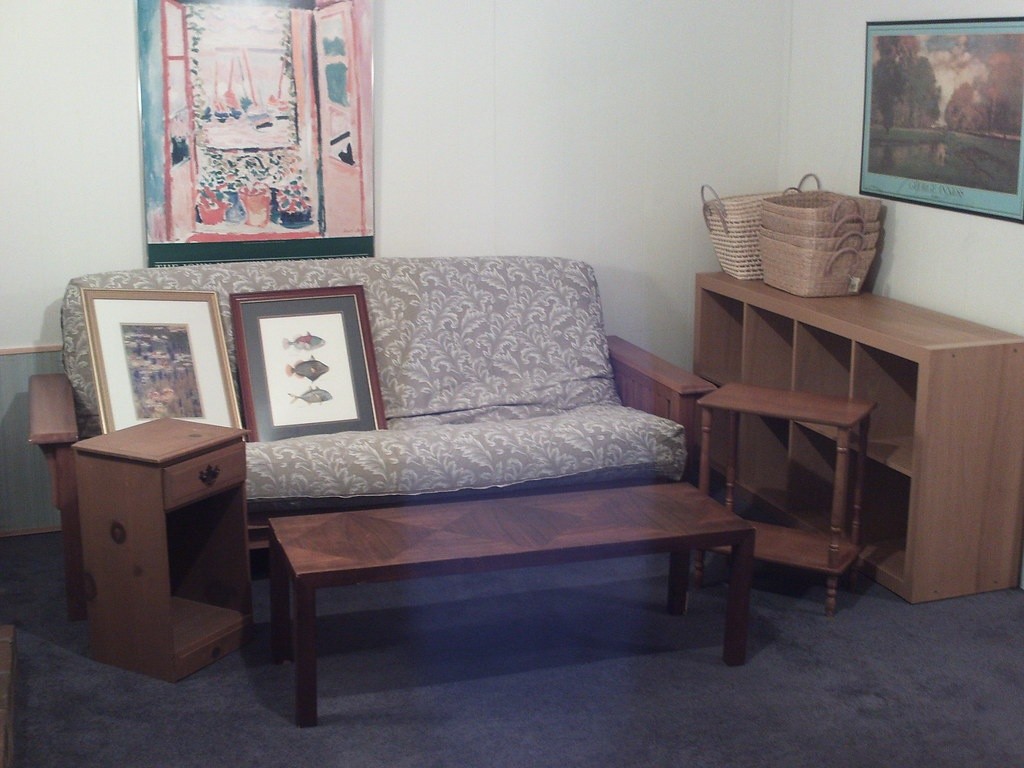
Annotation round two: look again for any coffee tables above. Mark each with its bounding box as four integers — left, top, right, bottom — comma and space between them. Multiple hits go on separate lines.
267, 482, 757, 728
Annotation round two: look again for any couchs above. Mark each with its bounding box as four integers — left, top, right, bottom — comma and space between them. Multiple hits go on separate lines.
27, 255, 718, 623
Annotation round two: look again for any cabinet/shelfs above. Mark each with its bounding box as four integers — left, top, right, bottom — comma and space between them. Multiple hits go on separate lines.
71, 417, 255, 683
692, 270, 1024, 604
696, 382, 875, 618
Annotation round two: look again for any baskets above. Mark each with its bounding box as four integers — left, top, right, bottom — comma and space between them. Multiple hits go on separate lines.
702, 172, 882, 297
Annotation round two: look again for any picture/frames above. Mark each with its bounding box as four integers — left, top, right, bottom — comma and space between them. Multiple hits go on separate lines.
80, 287, 248, 442
230, 284, 387, 442
859, 16, 1024, 226
